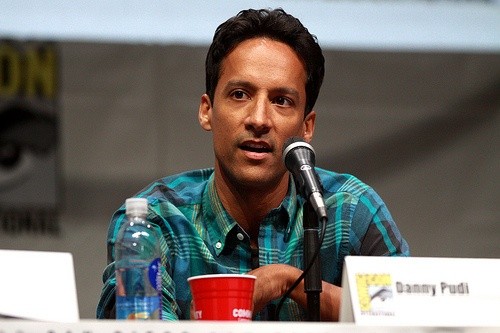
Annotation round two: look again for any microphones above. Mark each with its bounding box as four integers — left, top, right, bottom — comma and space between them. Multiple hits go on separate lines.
282, 136, 328, 223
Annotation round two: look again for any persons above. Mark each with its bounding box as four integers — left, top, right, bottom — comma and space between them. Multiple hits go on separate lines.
96, 7, 410, 323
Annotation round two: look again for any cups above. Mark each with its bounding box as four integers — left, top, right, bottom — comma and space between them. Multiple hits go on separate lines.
187, 274, 257, 320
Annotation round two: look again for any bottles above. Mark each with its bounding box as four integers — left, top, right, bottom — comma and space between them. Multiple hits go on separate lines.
115, 198, 162, 320
